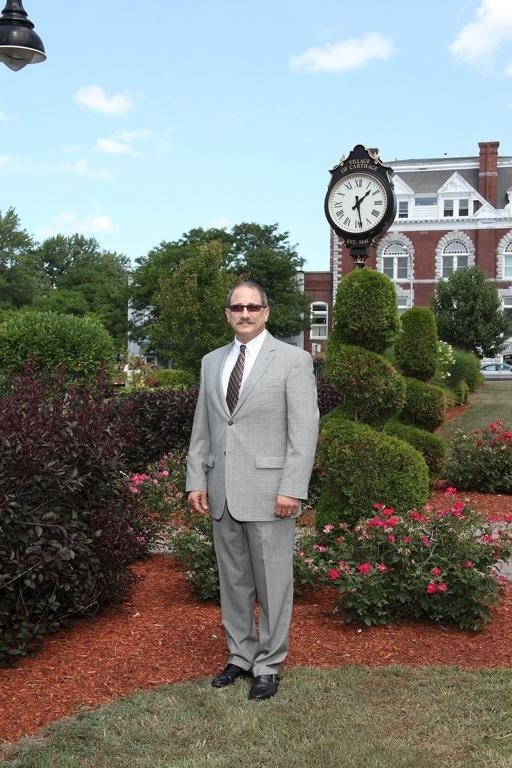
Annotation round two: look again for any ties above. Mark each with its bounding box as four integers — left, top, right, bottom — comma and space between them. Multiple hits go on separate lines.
226, 344, 246, 415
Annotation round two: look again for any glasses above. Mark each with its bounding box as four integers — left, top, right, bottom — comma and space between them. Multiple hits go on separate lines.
229, 304, 267, 313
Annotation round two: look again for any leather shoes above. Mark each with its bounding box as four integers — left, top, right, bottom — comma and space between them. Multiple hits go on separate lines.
250, 674, 279, 698
212, 663, 250, 688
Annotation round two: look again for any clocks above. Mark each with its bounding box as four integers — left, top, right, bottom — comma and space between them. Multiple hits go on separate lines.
324, 144, 397, 247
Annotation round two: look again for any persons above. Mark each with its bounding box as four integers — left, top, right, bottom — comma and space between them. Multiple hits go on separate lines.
184, 277, 324, 700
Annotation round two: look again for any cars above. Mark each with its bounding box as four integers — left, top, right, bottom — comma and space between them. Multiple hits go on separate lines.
481, 363, 512, 380
